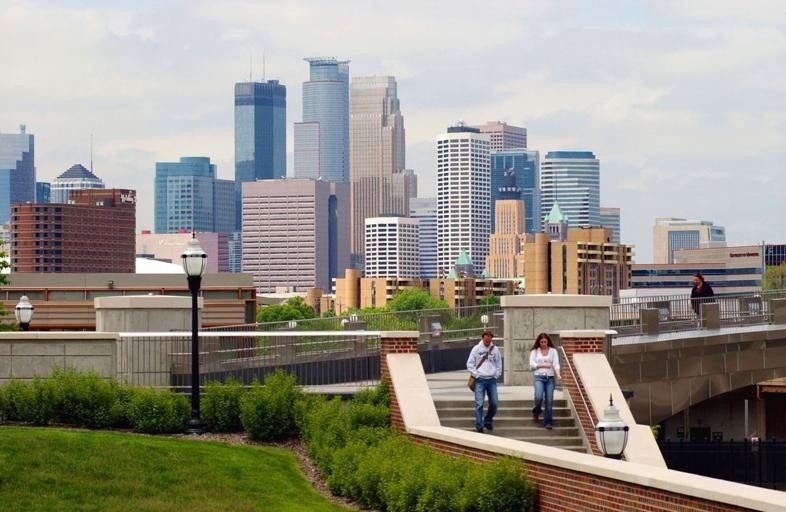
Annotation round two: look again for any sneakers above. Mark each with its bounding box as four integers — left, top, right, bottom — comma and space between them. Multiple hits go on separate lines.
532, 414, 554, 430
475, 418, 494, 434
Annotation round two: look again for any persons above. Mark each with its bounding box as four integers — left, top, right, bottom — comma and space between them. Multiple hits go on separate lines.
528, 331, 563, 431
689, 271, 715, 331
466, 329, 502, 432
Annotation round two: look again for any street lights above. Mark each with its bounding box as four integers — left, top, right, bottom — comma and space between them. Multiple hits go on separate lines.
181, 233, 208, 434
15, 290, 35, 331
594, 392, 629, 459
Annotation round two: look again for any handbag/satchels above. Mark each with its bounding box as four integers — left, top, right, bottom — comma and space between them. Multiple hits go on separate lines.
468, 376, 477, 391
